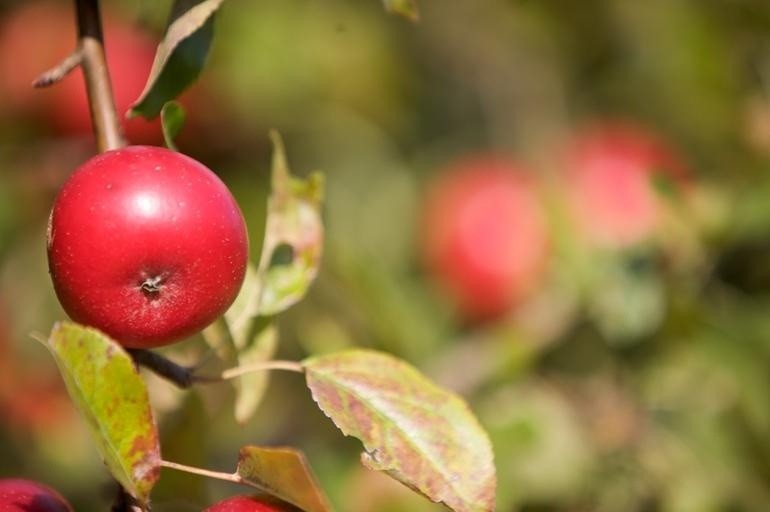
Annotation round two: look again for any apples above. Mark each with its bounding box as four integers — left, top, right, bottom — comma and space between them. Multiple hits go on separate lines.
0, 4, 75, 114
0, 478, 72, 512
425, 157, 545, 315
55, 22, 195, 144
204, 495, 297, 512
46, 145, 249, 349
574, 120, 694, 240
